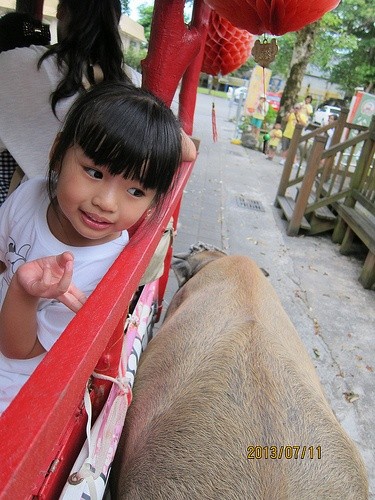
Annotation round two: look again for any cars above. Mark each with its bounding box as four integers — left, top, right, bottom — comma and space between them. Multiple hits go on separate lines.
313, 105, 341, 126
258, 92, 282, 113
317, 98, 347, 108
234, 87, 248, 102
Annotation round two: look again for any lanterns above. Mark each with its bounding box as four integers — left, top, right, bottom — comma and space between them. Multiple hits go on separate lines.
199, 7, 253, 142
201, 1, 338, 156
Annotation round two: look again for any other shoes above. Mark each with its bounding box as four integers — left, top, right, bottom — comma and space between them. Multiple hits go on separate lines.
280, 159, 285, 165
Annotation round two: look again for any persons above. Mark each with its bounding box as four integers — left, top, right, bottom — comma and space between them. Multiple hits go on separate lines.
0, 80, 182, 417
279, 94, 313, 168
251, 93, 269, 143
265, 121, 282, 162
323, 114, 338, 151
0, 0, 197, 182
